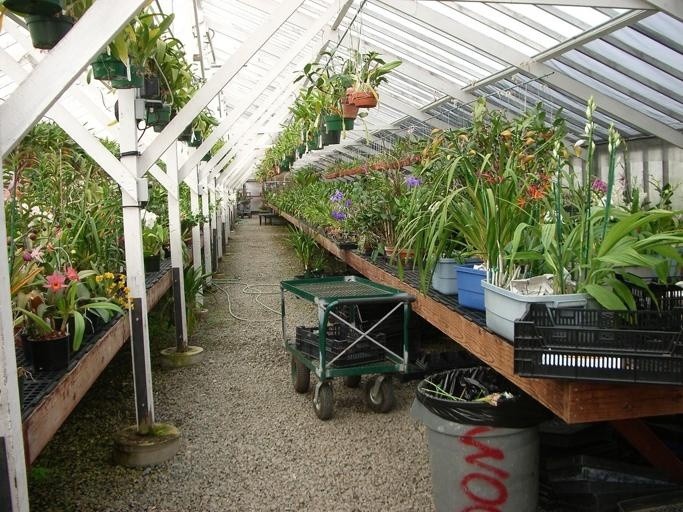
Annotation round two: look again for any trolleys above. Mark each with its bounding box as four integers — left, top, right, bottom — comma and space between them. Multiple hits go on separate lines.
277, 273, 418, 420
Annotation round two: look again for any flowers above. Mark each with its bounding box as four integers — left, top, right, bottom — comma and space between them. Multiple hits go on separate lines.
13, 265, 135, 353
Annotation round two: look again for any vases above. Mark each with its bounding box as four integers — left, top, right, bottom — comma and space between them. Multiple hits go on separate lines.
22, 331, 71, 372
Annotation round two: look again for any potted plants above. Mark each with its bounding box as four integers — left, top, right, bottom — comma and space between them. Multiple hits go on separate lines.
253, 46, 405, 182
1, 173, 219, 270
258, 182, 366, 300
163, 263, 218, 336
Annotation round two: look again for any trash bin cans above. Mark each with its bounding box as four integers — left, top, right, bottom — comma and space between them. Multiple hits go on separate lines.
410, 367, 555, 512
